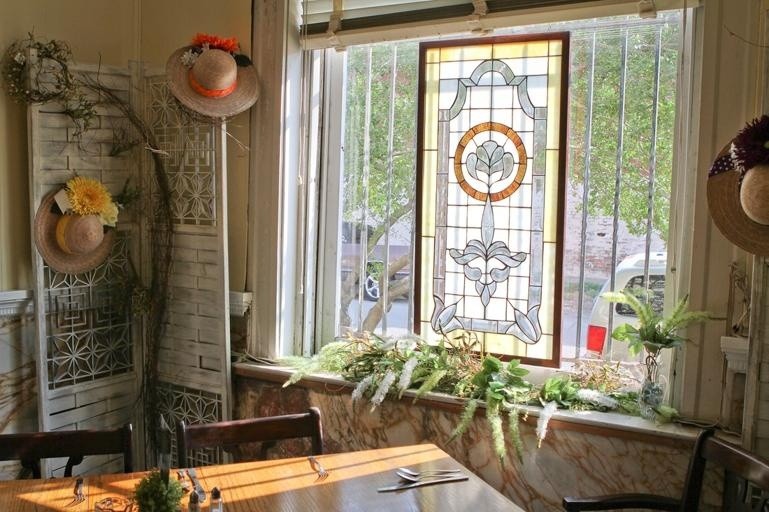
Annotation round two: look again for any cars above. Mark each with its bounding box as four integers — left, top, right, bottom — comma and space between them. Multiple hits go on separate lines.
341, 207, 413, 302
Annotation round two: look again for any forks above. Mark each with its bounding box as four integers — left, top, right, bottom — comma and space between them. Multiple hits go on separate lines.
77, 478, 84, 503
309, 457, 329, 478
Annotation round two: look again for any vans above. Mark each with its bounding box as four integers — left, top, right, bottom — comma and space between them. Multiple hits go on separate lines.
584, 251, 667, 388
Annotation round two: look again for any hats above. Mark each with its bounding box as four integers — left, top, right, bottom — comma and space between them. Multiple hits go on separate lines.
166, 46, 261, 117
706, 134, 769, 258
34, 186, 117, 276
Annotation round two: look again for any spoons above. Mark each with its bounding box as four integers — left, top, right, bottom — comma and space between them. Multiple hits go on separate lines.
395, 466, 464, 483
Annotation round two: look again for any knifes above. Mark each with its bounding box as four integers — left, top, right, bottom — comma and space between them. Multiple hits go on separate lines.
377, 476, 469, 492
187, 469, 206, 502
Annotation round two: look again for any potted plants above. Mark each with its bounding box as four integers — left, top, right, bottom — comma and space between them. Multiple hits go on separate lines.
598, 286, 712, 421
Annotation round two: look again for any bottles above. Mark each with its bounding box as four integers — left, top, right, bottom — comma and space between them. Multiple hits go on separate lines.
188, 487, 223, 511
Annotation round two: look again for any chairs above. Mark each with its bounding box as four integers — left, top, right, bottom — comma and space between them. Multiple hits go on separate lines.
562, 428, 769, 512
176, 407, 323, 468
0, 423, 133, 480
